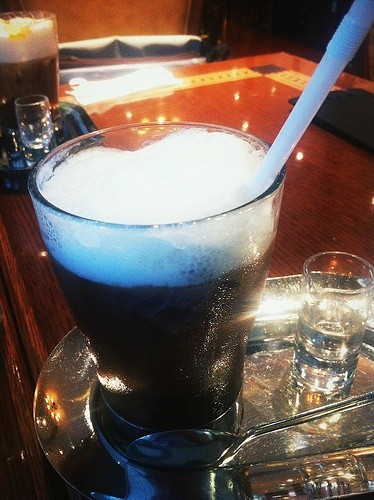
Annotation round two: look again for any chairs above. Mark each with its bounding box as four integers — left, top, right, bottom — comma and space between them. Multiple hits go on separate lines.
0, 0, 230, 70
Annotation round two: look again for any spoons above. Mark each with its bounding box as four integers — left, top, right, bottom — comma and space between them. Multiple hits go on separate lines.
125, 391, 374, 474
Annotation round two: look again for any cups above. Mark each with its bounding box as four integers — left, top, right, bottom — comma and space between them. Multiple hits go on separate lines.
0, 11, 63, 133
291, 250, 374, 392
15, 94, 53, 150
28, 121, 287, 460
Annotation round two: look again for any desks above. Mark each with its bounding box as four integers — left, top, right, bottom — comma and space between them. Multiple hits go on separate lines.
0, 51, 374, 500
